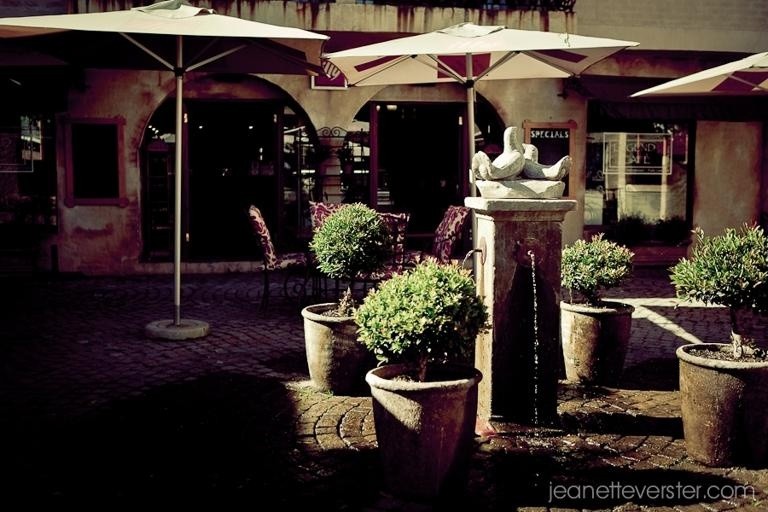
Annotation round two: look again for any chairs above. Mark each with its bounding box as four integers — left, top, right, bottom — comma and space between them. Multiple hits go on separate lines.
245, 201, 471, 325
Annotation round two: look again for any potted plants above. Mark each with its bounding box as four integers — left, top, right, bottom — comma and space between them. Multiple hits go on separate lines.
665, 218, 768, 469
349, 251, 495, 512
300, 200, 396, 398
557, 231, 637, 388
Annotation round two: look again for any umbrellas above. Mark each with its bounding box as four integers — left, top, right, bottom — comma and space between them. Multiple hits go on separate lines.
320, 20, 640, 273
0, 0, 330, 326
626, 50, 768, 99
